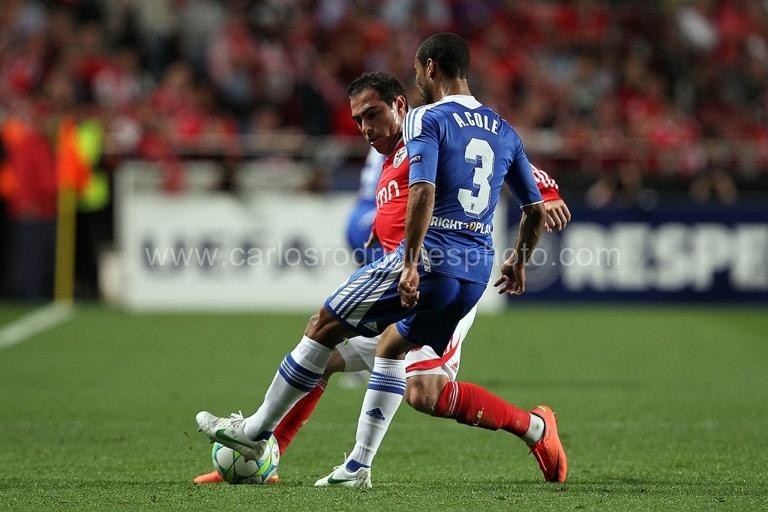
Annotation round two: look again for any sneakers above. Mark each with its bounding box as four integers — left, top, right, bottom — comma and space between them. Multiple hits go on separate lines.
195, 410, 268, 461
314, 453, 372, 489
191, 470, 280, 484
526, 403, 567, 483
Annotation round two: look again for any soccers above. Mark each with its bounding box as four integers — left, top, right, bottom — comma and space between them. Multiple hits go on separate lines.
212, 433, 280, 484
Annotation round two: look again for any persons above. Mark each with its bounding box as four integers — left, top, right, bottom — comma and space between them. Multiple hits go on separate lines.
0, 0, 767, 490
